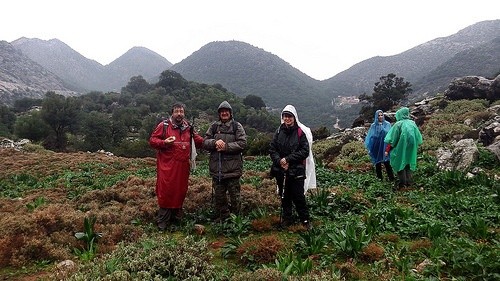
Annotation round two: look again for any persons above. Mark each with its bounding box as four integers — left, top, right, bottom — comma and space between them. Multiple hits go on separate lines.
204, 100, 247, 223
149, 103, 203, 230
363, 109, 395, 181
384, 108, 423, 190
269, 105, 317, 227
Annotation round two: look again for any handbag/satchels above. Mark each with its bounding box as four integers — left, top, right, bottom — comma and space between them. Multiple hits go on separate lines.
297, 128, 307, 168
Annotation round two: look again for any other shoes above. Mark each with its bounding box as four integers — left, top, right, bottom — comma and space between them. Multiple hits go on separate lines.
214, 216, 225, 223
397, 186, 412, 191
376, 177, 382, 182
175, 216, 188, 222
156, 222, 167, 230
302, 220, 314, 230
389, 177, 396, 181
280, 218, 296, 228
